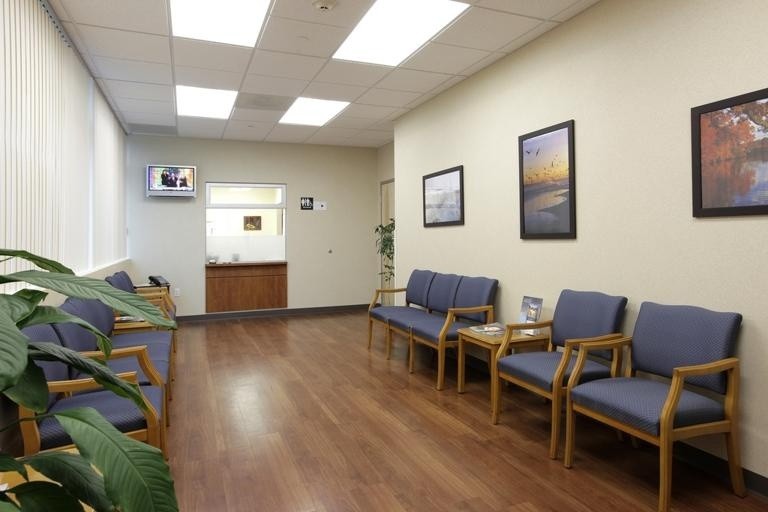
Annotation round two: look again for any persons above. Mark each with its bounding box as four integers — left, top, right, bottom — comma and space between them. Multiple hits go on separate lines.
160, 170, 188, 188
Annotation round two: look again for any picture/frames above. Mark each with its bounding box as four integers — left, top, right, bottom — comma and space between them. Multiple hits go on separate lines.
422, 165, 465, 228
691, 88, 768, 217
517, 119, 577, 240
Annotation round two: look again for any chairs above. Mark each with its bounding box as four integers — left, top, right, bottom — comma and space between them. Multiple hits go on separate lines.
408, 275, 498, 390
366, 268, 437, 360
565, 301, 743, 511
104, 270, 177, 322
51, 304, 170, 423
68, 295, 174, 380
492, 290, 628, 460
10, 323, 168, 471
386, 272, 463, 373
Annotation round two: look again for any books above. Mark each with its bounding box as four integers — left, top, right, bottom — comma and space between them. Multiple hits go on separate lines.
473, 296, 543, 337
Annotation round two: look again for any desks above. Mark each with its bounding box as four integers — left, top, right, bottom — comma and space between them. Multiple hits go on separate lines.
112, 311, 166, 335
455, 322, 550, 411
0, 458, 97, 511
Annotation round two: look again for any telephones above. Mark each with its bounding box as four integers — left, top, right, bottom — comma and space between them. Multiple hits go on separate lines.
148, 275, 171, 287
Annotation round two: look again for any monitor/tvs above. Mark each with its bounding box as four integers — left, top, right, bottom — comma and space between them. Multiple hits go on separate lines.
146, 165, 196, 198
